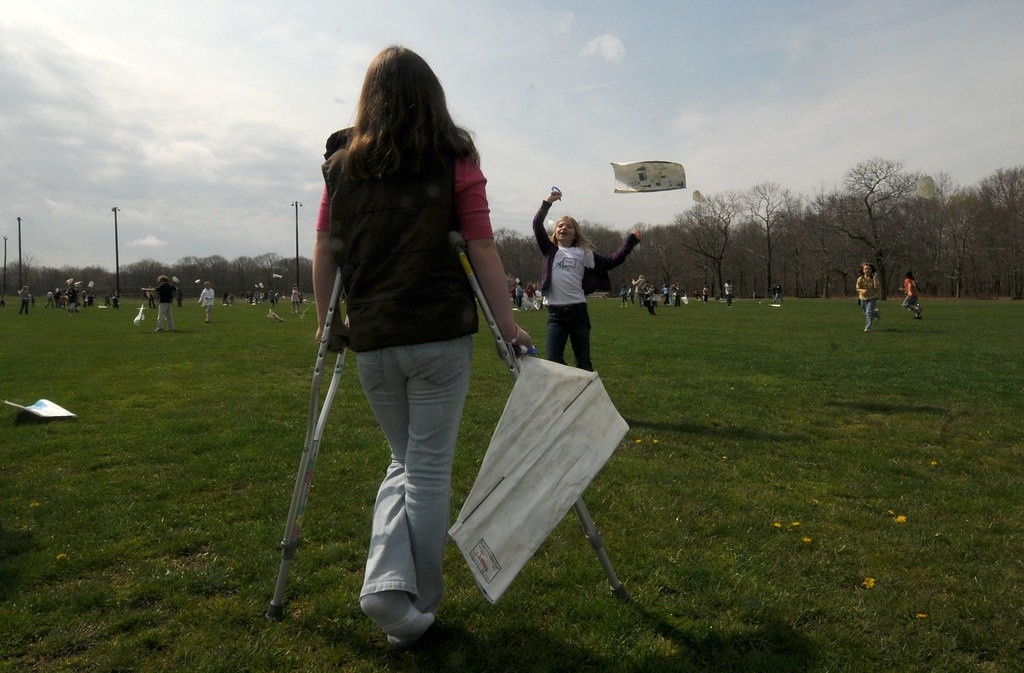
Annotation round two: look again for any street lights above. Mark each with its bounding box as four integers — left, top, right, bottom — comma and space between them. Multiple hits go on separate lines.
3, 235, 8, 296
290, 200, 303, 303
111, 206, 121, 299
16, 216, 23, 293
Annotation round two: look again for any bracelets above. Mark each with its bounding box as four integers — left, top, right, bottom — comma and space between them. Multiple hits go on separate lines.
511, 324, 520, 343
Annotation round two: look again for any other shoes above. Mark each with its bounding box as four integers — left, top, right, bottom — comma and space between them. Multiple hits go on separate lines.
864, 329, 871, 332
913, 316, 922, 320
154, 328, 163, 332
916, 306, 922, 316
874, 308, 880, 321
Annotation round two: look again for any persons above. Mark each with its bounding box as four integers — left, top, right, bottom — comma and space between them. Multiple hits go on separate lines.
141, 275, 177, 332
240, 290, 278, 305
19, 286, 30, 314
147, 286, 156, 308
45, 287, 96, 314
620, 274, 658, 314
724, 280, 735, 306
771, 284, 781, 298
290, 283, 300, 314
31, 295, 35, 306
856, 263, 880, 332
197, 282, 214, 322
104, 288, 119, 309
692, 285, 709, 304
511, 281, 536, 308
533, 192, 641, 373
177, 292, 183, 308
222, 289, 234, 305
898, 271, 922, 319
662, 282, 681, 307
312, 46, 534, 643
0, 291, 8, 308
